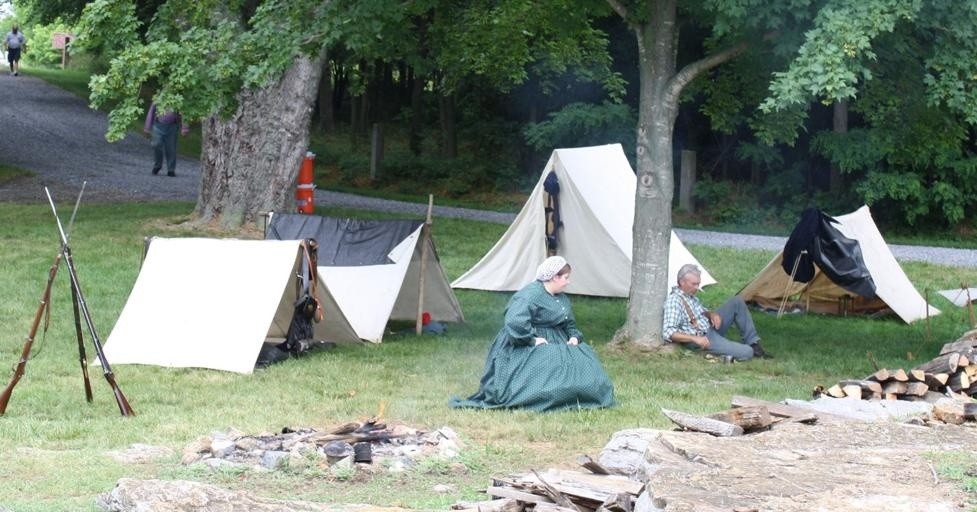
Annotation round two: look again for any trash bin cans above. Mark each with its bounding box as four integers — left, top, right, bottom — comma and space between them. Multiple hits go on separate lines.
296, 152, 316, 214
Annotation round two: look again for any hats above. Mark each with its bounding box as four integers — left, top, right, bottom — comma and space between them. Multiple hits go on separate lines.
535, 255, 567, 282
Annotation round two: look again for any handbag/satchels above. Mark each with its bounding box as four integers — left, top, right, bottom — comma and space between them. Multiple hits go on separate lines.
312, 295, 326, 323
294, 294, 318, 324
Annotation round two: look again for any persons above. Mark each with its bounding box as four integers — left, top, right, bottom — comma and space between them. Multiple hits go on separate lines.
144, 101, 190, 177
6, 23, 28, 76
448, 255, 615, 413
661, 263, 775, 362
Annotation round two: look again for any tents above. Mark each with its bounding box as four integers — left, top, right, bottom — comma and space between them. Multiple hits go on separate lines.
262, 211, 466, 344
736, 204, 944, 326
90, 236, 365, 374
446, 142, 718, 298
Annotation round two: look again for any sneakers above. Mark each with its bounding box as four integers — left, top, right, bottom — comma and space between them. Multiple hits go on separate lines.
151, 167, 175, 178
751, 342, 774, 359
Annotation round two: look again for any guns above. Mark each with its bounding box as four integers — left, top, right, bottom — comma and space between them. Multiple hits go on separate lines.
1, 180, 87, 416
67, 244, 93, 404
44, 185, 135, 417
